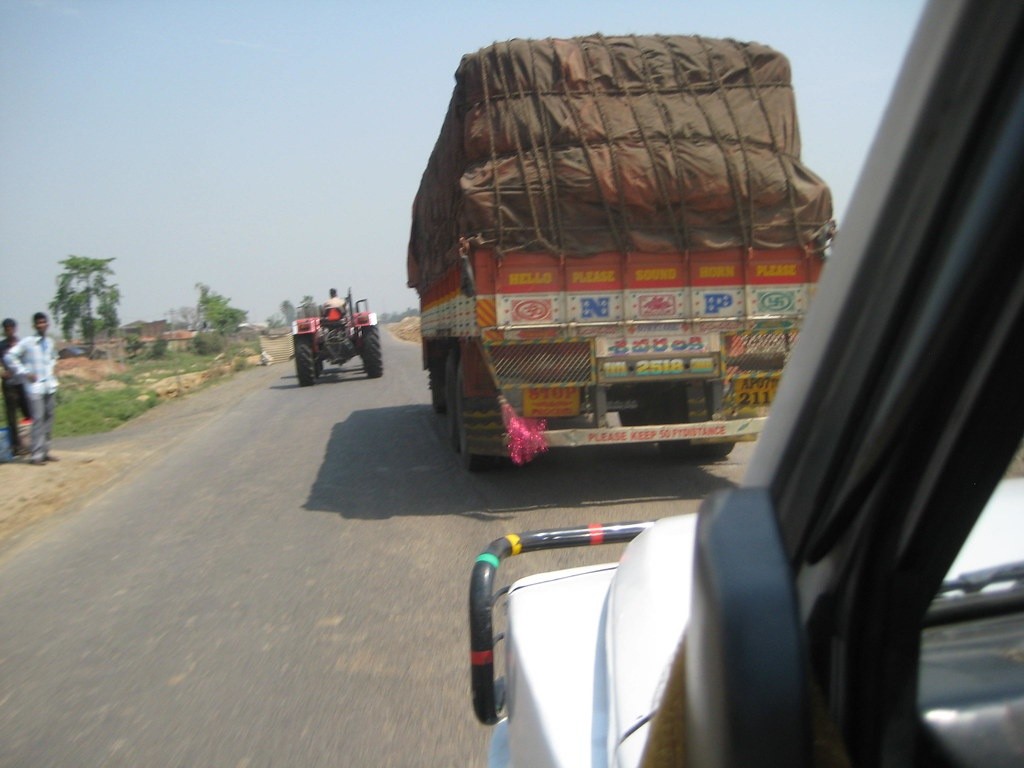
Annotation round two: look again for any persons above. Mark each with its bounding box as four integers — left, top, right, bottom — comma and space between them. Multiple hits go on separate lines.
324, 288, 347, 316
0, 313, 61, 465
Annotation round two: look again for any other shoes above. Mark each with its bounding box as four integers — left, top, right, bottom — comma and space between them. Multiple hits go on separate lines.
44, 456, 60, 462
29, 460, 46, 465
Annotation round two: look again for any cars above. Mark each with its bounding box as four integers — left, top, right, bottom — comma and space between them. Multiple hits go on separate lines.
57, 345, 88, 359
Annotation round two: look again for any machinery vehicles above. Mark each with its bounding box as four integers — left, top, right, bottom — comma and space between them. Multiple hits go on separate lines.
291, 286, 384, 387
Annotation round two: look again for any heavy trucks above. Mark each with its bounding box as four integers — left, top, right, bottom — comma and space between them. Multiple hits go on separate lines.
407, 31, 836, 471
